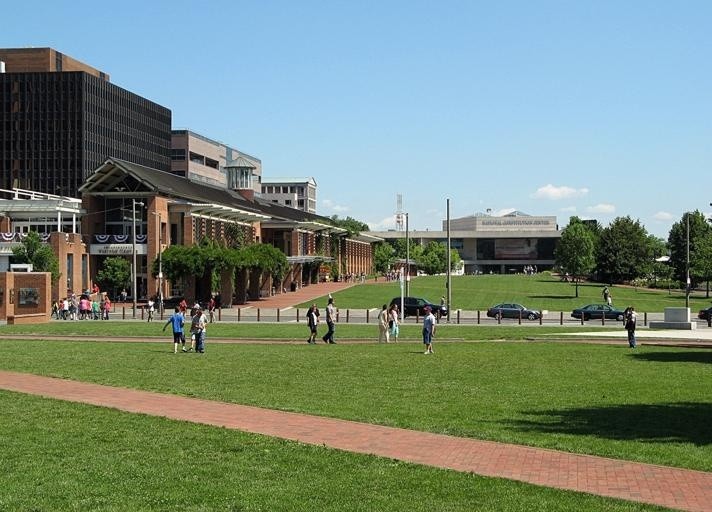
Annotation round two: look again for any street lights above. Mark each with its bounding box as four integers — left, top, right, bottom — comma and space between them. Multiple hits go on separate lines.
686, 213, 693, 306
133, 198, 145, 301
402, 213, 410, 263
151, 211, 162, 296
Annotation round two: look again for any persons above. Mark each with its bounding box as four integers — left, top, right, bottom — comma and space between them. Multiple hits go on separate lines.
163, 298, 216, 354
608, 295, 612, 306
83, 288, 89, 295
121, 288, 127, 302
148, 298, 155, 322
307, 299, 338, 344
523, 265, 537, 275
92, 283, 99, 294
68, 290, 76, 299
441, 296, 447, 309
51, 295, 111, 320
345, 271, 368, 283
422, 306, 436, 354
385, 270, 399, 282
602, 286, 610, 302
624, 306, 637, 349
378, 304, 401, 343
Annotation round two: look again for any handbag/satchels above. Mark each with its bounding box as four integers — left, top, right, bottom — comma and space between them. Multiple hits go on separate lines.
389, 321, 393, 328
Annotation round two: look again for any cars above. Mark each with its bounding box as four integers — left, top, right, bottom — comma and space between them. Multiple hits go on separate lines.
571, 304, 625, 321
698, 306, 712, 320
488, 303, 543, 321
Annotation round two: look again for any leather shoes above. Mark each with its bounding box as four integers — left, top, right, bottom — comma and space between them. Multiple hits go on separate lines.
329, 341, 337, 344
323, 337, 328, 343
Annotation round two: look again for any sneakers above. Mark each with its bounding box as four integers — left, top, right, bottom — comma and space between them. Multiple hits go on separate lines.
182, 348, 187, 352
424, 351, 430, 355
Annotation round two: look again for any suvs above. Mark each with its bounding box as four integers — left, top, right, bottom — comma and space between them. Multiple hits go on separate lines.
390, 298, 448, 320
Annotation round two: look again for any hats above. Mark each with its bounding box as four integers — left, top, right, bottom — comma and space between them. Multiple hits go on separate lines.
424, 306, 432, 310
